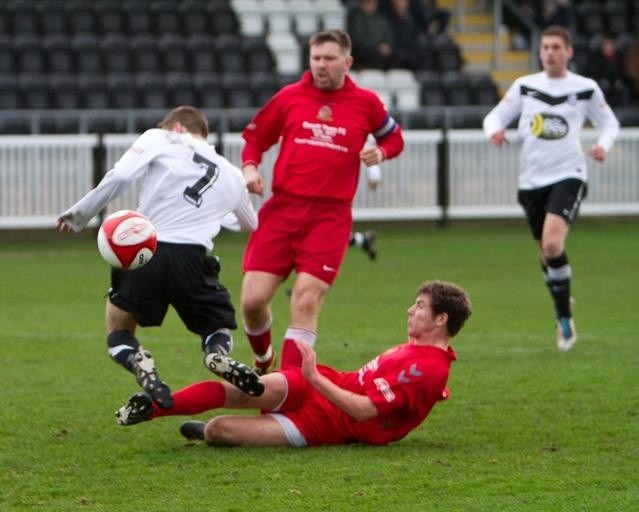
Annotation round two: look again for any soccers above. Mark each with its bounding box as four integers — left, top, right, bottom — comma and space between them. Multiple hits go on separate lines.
97, 210, 157, 270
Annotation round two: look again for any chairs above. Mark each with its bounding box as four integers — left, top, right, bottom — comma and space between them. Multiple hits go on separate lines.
2, 0, 519, 132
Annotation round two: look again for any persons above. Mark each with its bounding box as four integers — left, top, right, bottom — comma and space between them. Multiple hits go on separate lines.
238, 28, 406, 378
483, 24, 621, 352
353, 0, 638, 126
116, 278, 473, 448
56, 104, 264, 408
347, 230, 376, 259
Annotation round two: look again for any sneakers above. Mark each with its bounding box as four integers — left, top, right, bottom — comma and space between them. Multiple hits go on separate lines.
179, 422, 205, 441
115, 392, 152, 425
204, 352, 264, 396
251, 351, 277, 376
555, 317, 578, 350
130, 345, 173, 408
362, 229, 377, 260
